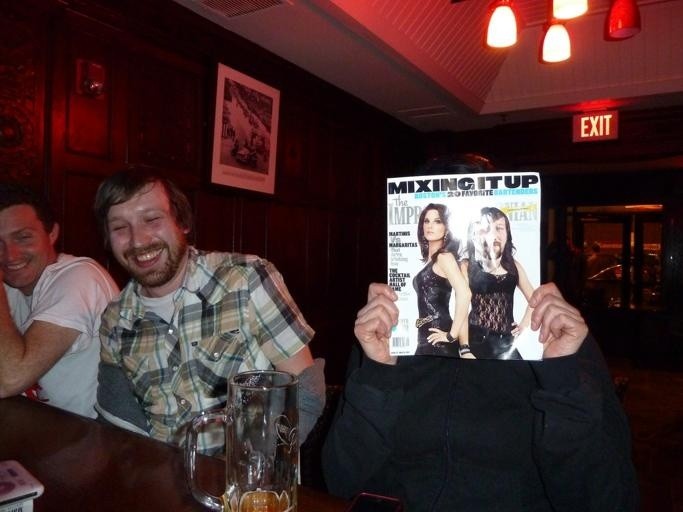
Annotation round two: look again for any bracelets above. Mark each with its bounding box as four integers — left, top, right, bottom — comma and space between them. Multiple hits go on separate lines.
458, 343, 472, 358
446, 331, 456, 344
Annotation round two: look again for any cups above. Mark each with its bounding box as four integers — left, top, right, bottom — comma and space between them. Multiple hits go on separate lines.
183, 370, 301, 512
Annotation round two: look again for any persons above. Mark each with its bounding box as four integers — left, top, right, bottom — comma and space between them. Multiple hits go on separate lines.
0, 187, 121, 420
456, 206, 536, 360
542, 240, 571, 289
584, 240, 609, 273
411, 202, 473, 357
320, 151, 641, 512
93, 162, 328, 467
231, 127, 256, 156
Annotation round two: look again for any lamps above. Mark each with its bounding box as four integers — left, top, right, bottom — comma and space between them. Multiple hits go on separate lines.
608, 1, 641, 40
542, 23, 572, 63
552, 0, 589, 22
486, 0, 518, 49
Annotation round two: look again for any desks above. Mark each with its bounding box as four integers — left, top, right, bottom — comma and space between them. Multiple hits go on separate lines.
1, 394, 399, 512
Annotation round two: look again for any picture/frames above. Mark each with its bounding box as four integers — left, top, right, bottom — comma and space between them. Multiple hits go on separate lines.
210, 63, 280, 195
